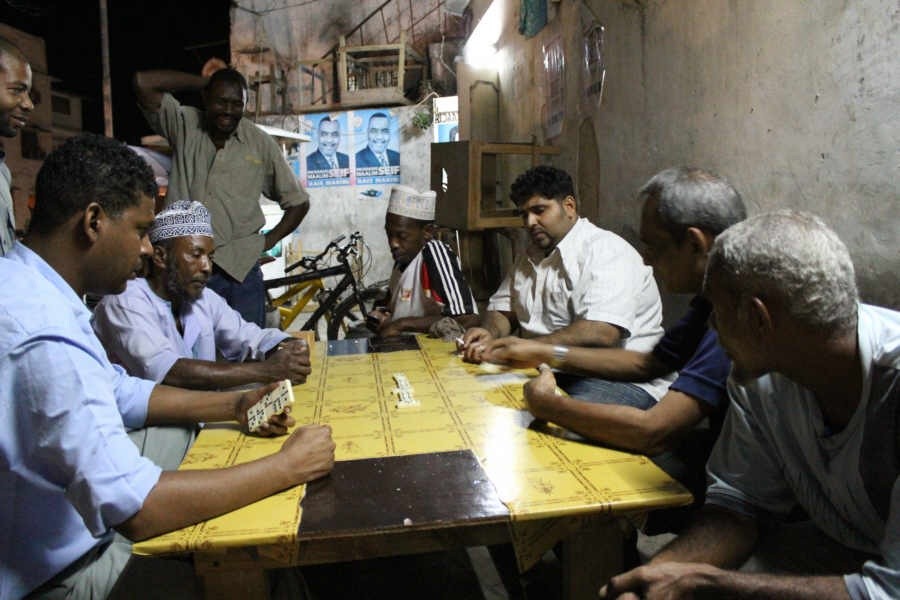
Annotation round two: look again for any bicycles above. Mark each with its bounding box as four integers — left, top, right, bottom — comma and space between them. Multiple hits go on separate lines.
264, 230, 385, 340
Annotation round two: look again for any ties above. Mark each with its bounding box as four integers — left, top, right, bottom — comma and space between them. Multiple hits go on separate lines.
330, 157, 337, 169
379, 155, 388, 166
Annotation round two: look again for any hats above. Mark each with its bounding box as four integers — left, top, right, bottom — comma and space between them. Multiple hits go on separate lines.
387, 185, 437, 221
150, 199, 215, 244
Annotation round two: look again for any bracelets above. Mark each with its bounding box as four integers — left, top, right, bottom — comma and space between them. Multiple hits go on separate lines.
265, 234, 272, 249
549, 344, 569, 369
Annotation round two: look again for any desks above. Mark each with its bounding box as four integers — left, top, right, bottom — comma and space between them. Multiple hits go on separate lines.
336, 31, 427, 106
430, 141, 562, 231
131, 329, 695, 600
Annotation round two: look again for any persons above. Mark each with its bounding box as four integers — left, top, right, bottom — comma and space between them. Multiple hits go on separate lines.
136, 59, 310, 330
0, 33, 35, 257
0, 135, 337, 600
456, 165, 666, 443
522, 165, 746, 600
94, 198, 312, 389
306, 116, 350, 169
343, 187, 481, 339
598, 207, 900, 600
356, 112, 399, 167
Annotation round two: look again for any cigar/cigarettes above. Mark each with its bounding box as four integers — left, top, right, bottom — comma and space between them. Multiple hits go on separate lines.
455, 338, 464, 344
